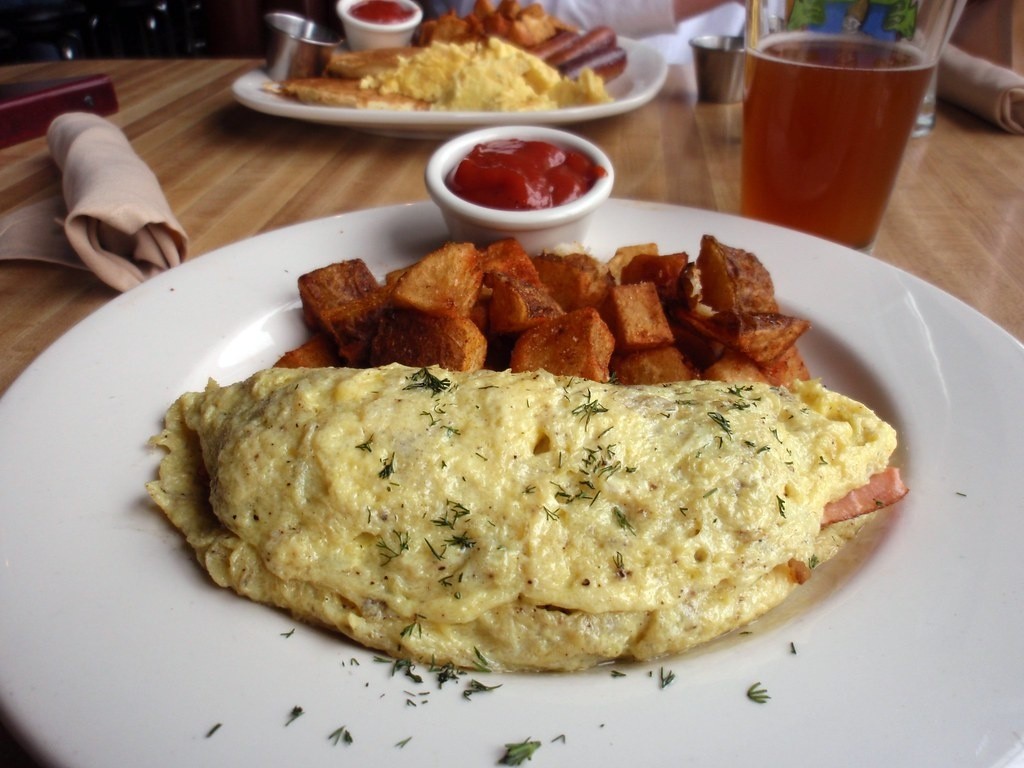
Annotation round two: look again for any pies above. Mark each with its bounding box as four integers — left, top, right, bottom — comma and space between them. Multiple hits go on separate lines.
257, 39, 445, 113
143, 364, 910, 674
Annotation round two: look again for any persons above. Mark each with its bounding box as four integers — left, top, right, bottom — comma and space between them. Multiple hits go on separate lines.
419, 1, 772, 93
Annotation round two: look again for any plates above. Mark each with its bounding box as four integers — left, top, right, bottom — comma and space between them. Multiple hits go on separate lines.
233, 32, 667, 139
2, 198, 1024, 768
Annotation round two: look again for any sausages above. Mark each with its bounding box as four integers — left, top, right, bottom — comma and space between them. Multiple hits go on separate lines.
531, 24, 627, 88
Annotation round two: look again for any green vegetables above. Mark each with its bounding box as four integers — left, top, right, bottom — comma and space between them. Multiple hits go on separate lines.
205, 366, 828, 768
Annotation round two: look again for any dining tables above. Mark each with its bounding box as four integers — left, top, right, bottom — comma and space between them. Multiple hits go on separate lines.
1, 58, 1024, 768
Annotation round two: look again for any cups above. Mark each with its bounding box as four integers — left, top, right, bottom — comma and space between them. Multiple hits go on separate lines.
740, 0, 965, 254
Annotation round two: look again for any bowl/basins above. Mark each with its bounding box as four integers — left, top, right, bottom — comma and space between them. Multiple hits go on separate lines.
425, 125, 615, 255
265, 9, 343, 84
335, 0, 423, 52
689, 36, 747, 103
